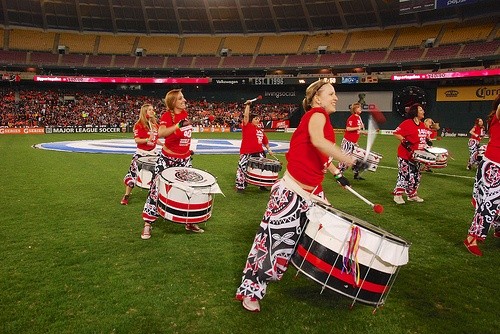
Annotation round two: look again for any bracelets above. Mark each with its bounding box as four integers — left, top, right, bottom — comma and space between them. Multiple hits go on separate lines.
177, 123, 180, 128
146, 138, 148, 142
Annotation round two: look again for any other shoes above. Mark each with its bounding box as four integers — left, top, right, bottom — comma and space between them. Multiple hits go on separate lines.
354, 175, 363, 181
426, 169, 434, 173
464, 237, 482, 257
467, 165, 471, 170
238, 189, 244, 193
260, 187, 270, 191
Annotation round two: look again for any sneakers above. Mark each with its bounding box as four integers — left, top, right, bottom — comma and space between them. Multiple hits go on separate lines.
186, 224, 204, 233
120, 195, 128, 204
141, 224, 151, 239
407, 196, 424, 202
242, 296, 261, 311
394, 195, 406, 204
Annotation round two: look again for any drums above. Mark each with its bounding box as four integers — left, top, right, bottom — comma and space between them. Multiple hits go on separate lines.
413, 150, 436, 164
156, 167, 217, 224
135, 157, 169, 189
478, 144, 488, 156
351, 144, 383, 172
289, 202, 413, 308
245, 157, 282, 187
424, 146, 448, 169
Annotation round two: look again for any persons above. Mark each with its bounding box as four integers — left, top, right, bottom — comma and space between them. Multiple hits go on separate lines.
236, 81, 369, 312
140, 89, 204, 239
466, 118, 485, 170
235, 95, 273, 191
121, 104, 164, 204
337, 102, 366, 181
393, 103, 431, 204
0, 88, 300, 128
425, 118, 440, 139
464, 94, 500, 256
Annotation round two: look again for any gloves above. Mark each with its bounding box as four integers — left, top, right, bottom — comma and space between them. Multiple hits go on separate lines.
178, 120, 189, 128
